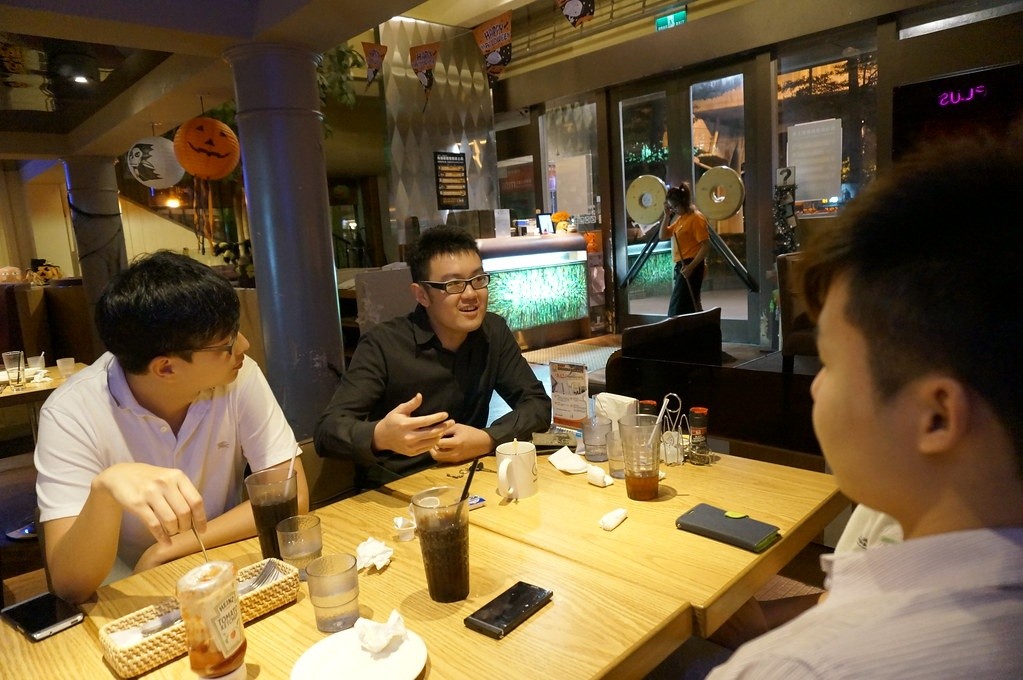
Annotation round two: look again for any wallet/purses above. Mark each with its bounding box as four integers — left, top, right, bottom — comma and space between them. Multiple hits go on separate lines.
532, 431, 577, 454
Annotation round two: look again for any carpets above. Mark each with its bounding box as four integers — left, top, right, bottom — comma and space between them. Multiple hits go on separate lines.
521, 341, 622, 373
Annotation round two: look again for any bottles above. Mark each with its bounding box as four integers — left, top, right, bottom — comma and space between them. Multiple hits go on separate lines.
688, 408, 709, 465
638, 400, 658, 416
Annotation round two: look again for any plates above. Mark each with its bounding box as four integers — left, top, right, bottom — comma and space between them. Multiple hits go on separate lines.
290, 627, 428, 680
0, 369, 47, 384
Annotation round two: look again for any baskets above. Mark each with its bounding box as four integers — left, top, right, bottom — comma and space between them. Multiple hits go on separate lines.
99, 560, 299, 678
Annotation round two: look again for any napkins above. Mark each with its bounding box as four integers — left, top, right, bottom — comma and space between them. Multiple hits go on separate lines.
355, 536, 393, 571
353, 609, 407, 654
548, 446, 592, 475
585, 466, 614, 486
599, 507, 627, 531
31, 373, 52, 382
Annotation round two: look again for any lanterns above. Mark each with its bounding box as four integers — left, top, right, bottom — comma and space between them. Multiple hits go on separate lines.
174, 117, 241, 180
127, 135, 185, 190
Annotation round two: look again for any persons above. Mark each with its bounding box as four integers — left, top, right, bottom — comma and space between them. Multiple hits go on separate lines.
658, 182, 711, 317
313, 224, 552, 492
702, 113, 1023, 680
34, 247, 309, 605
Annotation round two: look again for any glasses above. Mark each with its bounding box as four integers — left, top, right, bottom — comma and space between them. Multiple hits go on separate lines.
146, 320, 240, 364
418, 272, 490, 294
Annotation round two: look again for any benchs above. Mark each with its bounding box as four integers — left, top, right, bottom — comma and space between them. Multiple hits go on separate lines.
774, 251, 825, 371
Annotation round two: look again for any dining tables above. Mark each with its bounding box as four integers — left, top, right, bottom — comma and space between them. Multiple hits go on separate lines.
379, 440, 852, 642
0, 362, 91, 448
0, 488, 694, 680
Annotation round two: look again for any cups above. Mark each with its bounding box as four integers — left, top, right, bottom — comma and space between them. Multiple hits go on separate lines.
604, 430, 625, 479
275, 514, 322, 582
408, 497, 439, 522
173, 559, 248, 680
495, 441, 539, 499
411, 485, 469, 603
27, 356, 45, 370
245, 467, 299, 562
617, 413, 663, 500
395, 521, 417, 541
56, 357, 76, 379
2, 351, 27, 391
580, 415, 612, 462
306, 553, 360, 634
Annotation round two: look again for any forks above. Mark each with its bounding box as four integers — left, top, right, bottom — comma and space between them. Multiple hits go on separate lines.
141, 559, 276, 638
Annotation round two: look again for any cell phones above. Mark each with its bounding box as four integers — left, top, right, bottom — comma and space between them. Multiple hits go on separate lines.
463, 581, 553, 640
676, 503, 781, 552
2, 592, 84, 642
528, 432, 576, 456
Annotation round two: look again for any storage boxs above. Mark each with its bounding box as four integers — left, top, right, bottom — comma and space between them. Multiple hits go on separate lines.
592, 392, 640, 461
